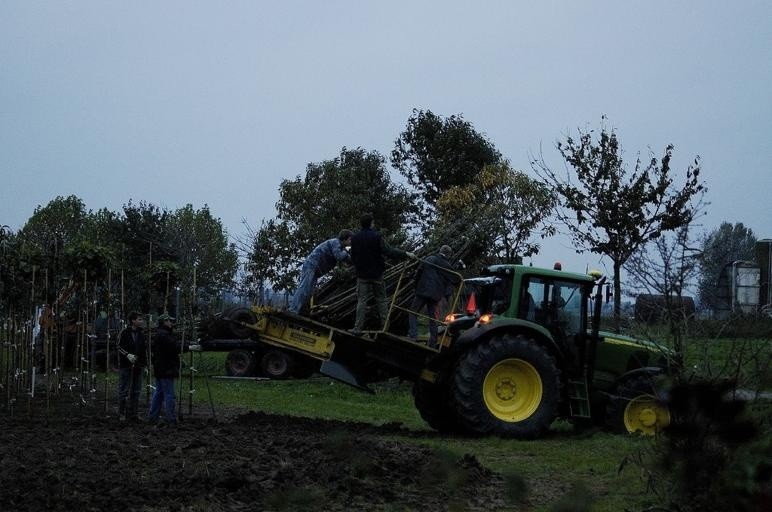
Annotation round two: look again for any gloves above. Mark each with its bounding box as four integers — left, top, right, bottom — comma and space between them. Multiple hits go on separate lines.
189, 345, 201, 351
127, 354, 138, 363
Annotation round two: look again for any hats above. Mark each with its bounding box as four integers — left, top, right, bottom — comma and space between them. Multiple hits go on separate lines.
440, 245, 452, 258
359, 214, 372, 225
157, 311, 176, 321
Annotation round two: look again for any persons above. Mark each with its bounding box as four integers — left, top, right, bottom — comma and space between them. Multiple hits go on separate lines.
147, 313, 201, 426
287, 229, 354, 316
115, 311, 147, 422
347, 213, 406, 335
503, 279, 538, 322
406, 245, 457, 350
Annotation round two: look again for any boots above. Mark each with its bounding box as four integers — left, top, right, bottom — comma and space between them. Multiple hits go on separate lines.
347, 320, 364, 334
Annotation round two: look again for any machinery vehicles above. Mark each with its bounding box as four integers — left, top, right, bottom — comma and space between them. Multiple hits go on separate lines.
247, 247, 682, 440
218, 254, 501, 385
